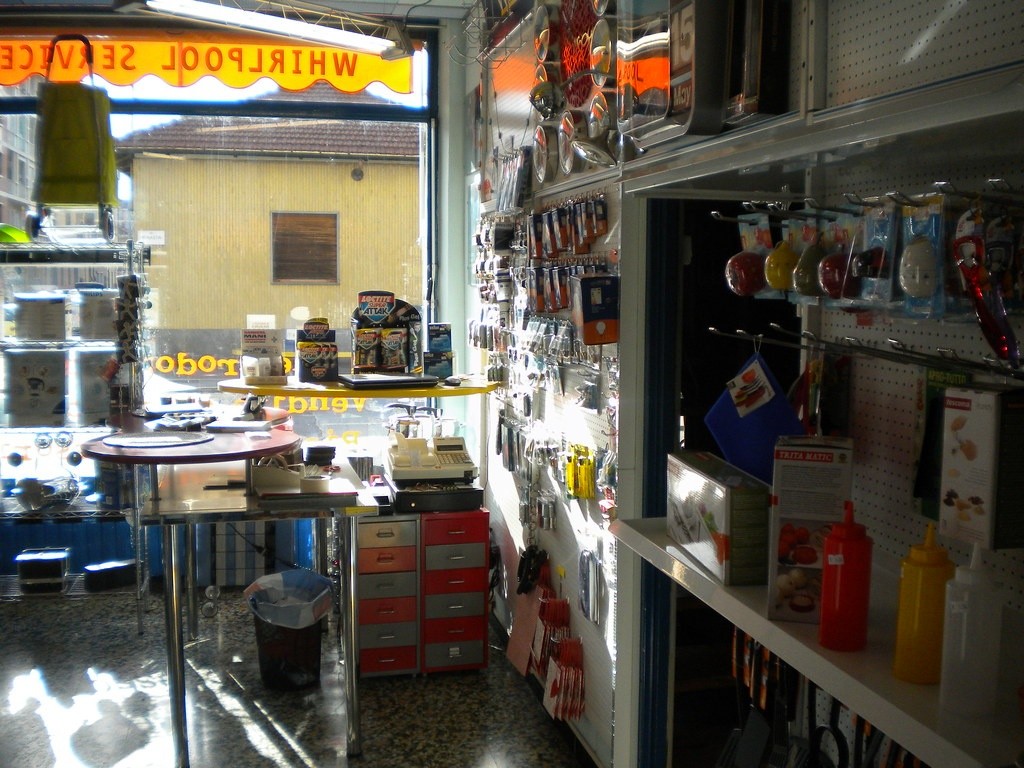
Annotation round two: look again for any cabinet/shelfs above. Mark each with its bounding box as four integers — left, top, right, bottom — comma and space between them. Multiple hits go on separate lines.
421, 506, 491, 677
0, 238, 151, 636
356, 507, 421, 678
609, 83, 1024, 768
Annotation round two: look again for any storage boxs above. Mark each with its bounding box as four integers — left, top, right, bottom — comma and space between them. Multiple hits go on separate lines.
768, 435, 854, 624
667, 451, 770, 587
939, 386, 1002, 551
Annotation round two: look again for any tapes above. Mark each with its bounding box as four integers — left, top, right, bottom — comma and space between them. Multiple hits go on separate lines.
298, 475, 331, 495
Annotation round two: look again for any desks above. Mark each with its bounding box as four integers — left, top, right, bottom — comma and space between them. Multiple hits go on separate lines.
220, 377, 498, 494
82, 429, 301, 638
137, 458, 378, 768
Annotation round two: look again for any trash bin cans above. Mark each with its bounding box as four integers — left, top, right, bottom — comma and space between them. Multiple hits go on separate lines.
246, 570, 331, 692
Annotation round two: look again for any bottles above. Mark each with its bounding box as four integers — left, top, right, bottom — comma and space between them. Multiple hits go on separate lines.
892, 521, 955, 685
939, 541, 1002, 715
817, 499, 875, 653
